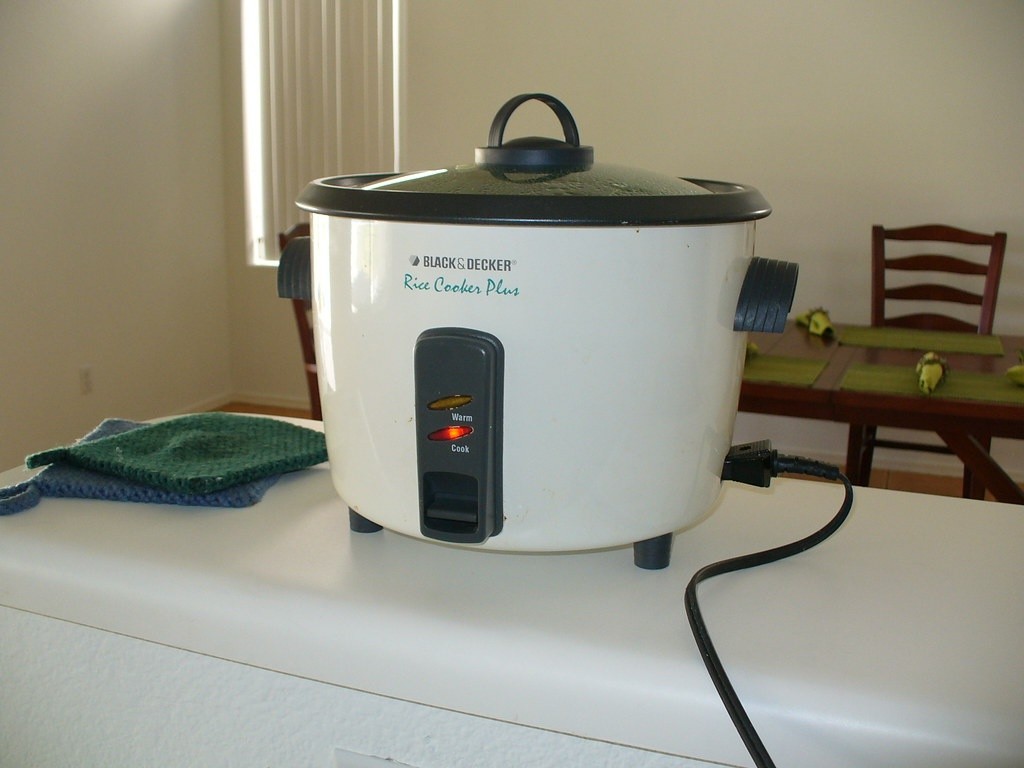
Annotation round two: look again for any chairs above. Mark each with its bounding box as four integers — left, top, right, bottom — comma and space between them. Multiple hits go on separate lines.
278, 222, 323, 421
845, 224, 1006, 501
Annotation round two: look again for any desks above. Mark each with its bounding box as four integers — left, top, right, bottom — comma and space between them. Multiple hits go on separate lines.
737, 320, 1024, 440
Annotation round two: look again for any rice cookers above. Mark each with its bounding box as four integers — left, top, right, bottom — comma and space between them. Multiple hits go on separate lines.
292, 92, 800, 573
